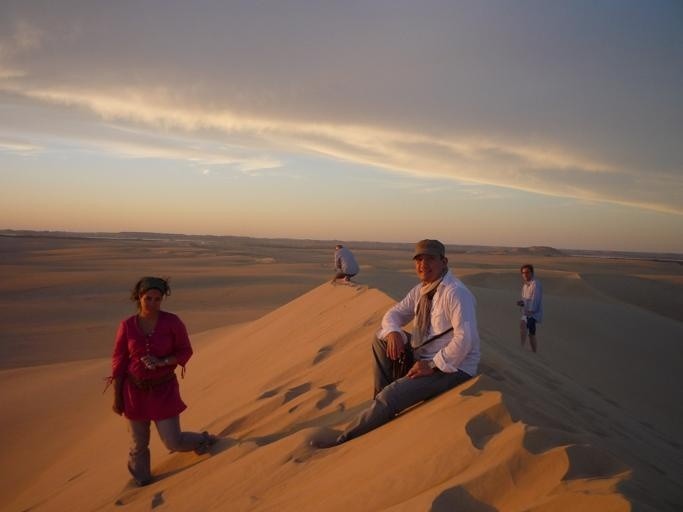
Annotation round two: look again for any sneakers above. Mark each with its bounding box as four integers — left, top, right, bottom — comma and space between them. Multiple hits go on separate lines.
310, 440, 340, 447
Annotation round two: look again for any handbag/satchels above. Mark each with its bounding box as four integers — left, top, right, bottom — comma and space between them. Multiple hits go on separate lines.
393, 350, 416, 377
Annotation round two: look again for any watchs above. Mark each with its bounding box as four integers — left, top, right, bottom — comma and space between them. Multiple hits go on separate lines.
160, 356, 169, 366
427, 360, 438, 369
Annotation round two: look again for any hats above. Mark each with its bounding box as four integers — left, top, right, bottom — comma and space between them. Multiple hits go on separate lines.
413, 239, 445, 259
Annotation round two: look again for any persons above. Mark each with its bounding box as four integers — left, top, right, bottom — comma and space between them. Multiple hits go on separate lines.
328, 239, 362, 284
308, 238, 481, 451
518, 262, 544, 354
107, 276, 216, 489
